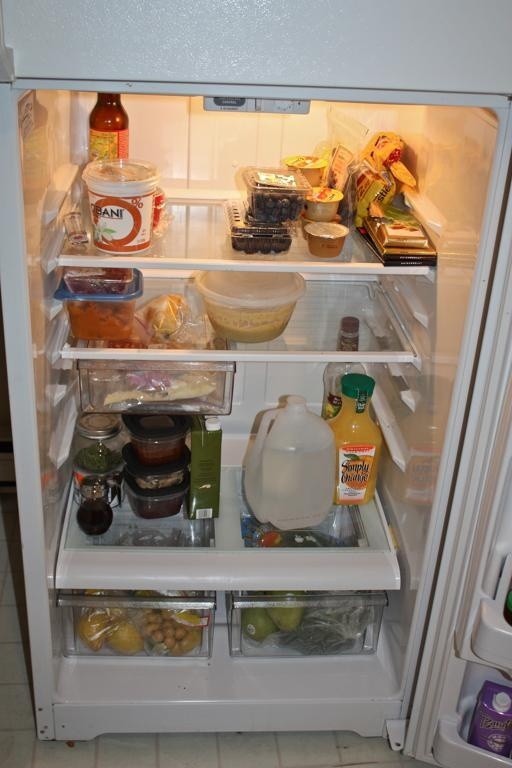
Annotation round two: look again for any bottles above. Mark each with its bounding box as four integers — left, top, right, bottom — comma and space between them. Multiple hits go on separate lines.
87, 84, 129, 179
466, 682, 511, 760
333, 375, 382, 508
185, 417, 222, 521
322, 314, 366, 424
71, 413, 127, 511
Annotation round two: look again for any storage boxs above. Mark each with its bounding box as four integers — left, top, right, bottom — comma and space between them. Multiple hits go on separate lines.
73, 358, 238, 415
225, 590, 390, 659
51, 588, 217, 658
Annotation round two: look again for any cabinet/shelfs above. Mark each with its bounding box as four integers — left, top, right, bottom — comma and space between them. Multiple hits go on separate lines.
41, 190, 427, 590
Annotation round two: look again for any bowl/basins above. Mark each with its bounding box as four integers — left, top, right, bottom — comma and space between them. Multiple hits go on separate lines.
198, 273, 304, 340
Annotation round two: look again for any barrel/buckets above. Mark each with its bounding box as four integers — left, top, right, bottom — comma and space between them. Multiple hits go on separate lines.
242, 395, 338, 532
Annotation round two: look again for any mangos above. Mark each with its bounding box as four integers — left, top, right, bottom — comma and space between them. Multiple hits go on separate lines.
106, 621, 151, 657
266, 589, 306, 633
77, 609, 108, 652
240, 605, 278, 644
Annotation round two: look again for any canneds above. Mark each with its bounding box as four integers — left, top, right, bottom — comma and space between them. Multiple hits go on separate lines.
81, 160, 161, 255
